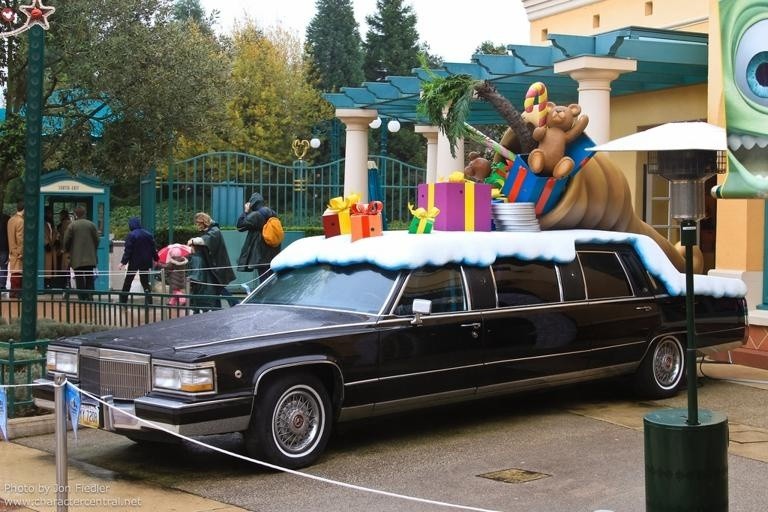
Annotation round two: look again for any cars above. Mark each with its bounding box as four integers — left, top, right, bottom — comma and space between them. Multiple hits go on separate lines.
33, 227, 749, 471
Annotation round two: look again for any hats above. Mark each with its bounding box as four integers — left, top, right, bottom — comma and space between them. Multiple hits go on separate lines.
171, 248, 181, 258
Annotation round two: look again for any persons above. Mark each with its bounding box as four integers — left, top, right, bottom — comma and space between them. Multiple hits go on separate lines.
0, 192, 281, 310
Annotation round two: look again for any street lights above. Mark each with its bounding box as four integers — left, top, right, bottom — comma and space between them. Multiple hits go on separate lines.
369, 116, 401, 230
310, 117, 344, 198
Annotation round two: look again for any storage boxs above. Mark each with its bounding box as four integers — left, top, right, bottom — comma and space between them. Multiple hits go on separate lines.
499, 131, 598, 215
320, 172, 494, 242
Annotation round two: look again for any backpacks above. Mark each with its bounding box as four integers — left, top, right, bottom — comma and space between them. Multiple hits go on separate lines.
260, 207, 285, 248
